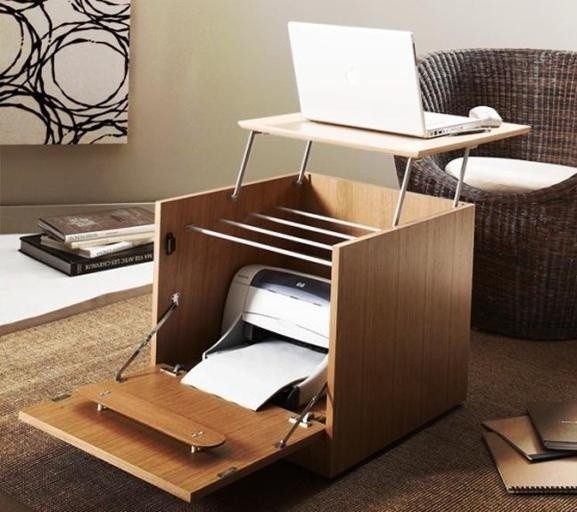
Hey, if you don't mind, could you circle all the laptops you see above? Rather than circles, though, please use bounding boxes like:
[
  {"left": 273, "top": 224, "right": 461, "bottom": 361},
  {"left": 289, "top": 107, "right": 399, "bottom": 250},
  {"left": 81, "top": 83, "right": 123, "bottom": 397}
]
[{"left": 285, "top": 20, "right": 476, "bottom": 139}]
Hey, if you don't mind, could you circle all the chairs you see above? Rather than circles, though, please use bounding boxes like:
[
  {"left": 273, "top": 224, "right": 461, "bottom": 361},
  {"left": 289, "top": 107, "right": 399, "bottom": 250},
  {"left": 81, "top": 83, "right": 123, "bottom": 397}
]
[{"left": 394, "top": 48, "right": 576, "bottom": 343}]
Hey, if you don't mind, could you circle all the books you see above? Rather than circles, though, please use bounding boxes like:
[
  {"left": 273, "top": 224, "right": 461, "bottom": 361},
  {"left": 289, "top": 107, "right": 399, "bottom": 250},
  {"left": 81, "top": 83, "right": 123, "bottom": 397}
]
[
  {"left": 18, "top": 206, "right": 154, "bottom": 276},
  {"left": 480, "top": 400, "right": 577, "bottom": 495}
]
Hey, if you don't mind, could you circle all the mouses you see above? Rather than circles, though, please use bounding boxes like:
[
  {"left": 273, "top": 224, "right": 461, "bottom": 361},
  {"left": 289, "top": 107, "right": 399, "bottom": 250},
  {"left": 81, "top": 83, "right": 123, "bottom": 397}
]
[{"left": 468, "top": 103, "right": 505, "bottom": 129}]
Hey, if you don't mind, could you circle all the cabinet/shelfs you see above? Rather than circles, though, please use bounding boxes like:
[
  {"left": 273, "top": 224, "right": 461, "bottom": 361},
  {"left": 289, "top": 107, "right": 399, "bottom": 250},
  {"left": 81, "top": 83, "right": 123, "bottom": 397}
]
[{"left": 19, "top": 104, "right": 531, "bottom": 504}]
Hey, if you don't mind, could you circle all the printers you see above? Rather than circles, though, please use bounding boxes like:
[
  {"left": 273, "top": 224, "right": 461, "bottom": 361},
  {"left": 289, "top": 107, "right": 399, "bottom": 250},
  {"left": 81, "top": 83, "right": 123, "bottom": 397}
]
[{"left": 183, "top": 261, "right": 331, "bottom": 412}]
